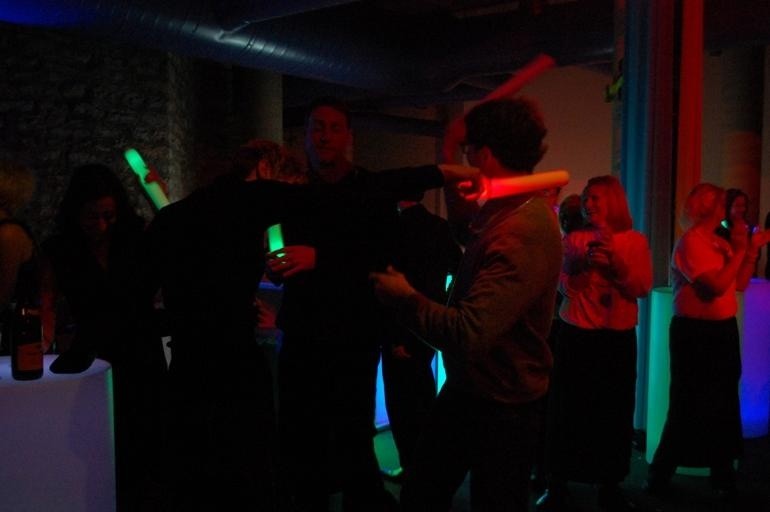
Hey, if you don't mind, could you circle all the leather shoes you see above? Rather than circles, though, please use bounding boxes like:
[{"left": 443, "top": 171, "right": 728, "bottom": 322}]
[
  {"left": 380, "top": 466, "right": 403, "bottom": 484},
  {"left": 535, "top": 489, "right": 550, "bottom": 512}
]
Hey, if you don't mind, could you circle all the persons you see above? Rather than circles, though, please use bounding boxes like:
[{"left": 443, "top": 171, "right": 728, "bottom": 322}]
[
  {"left": 640, "top": 181, "right": 768, "bottom": 497},
  {"left": 720, "top": 184, "right": 769, "bottom": 282}
]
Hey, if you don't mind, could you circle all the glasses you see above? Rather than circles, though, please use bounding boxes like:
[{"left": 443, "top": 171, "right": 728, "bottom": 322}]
[{"left": 461, "top": 138, "right": 480, "bottom": 154}]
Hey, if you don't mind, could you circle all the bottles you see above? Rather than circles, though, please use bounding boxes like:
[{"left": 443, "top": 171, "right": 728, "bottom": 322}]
[{"left": 8, "top": 261, "right": 45, "bottom": 380}]
